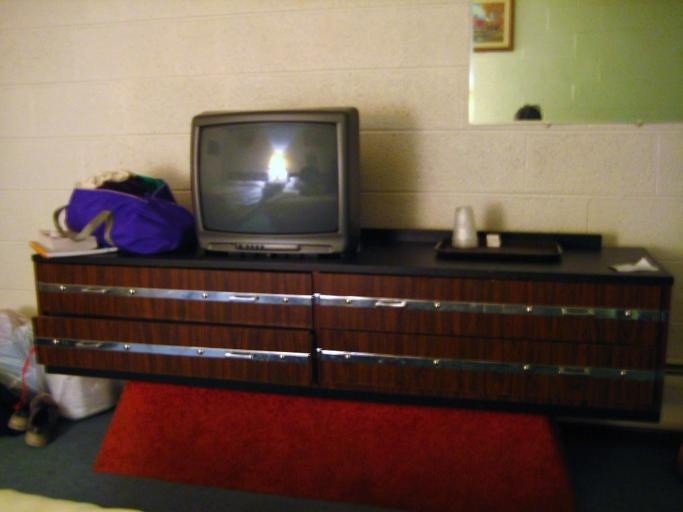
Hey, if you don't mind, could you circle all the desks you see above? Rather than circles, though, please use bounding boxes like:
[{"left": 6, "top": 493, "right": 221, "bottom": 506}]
[{"left": 30, "top": 247, "right": 675, "bottom": 423}]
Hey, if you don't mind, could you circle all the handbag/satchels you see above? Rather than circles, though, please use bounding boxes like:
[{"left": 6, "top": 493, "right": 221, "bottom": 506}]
[{"left": 53, "top": 176, "right": 196, "bottom": 258}]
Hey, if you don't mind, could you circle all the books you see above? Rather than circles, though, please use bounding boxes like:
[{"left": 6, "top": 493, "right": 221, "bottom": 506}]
[{"left": 26, "top": 226, "right": 121, "bottom": 261}]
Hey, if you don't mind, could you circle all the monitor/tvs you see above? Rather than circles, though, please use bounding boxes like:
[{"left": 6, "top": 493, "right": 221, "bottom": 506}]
[{"left": 191, "top": 107, "right": 360, "bottom": 255}]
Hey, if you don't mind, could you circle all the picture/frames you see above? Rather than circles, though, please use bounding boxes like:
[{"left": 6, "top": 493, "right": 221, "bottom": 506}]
[{"left": 471, "top": 1, "right": 514, "bottom": 51}]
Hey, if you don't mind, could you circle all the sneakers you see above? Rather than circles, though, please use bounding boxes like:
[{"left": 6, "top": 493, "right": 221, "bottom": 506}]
[
  {"left": 25, "top": 392, "right": 58, "bottom": 447},
  {"left": 8, "top": 401, "right": 30, "bottom": 432}
]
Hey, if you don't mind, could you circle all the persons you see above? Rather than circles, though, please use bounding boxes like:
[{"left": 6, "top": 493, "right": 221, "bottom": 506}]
[{"left": 514, "top": 102, "right": 543, "bottom": 122}]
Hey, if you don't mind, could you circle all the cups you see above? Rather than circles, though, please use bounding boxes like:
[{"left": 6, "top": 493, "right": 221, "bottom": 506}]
[{"left": 451, "top": 206, "right": 479, "bottom": 248}]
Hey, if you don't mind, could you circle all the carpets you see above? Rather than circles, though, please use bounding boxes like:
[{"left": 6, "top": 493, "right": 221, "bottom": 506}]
[{"left": 89, "top": 381, "right": 576, "bottom": 512}]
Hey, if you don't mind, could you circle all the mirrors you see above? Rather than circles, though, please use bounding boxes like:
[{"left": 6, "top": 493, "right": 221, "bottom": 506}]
[{"left": 469, "top": 0, "right": 682, "bottom": 123}]
